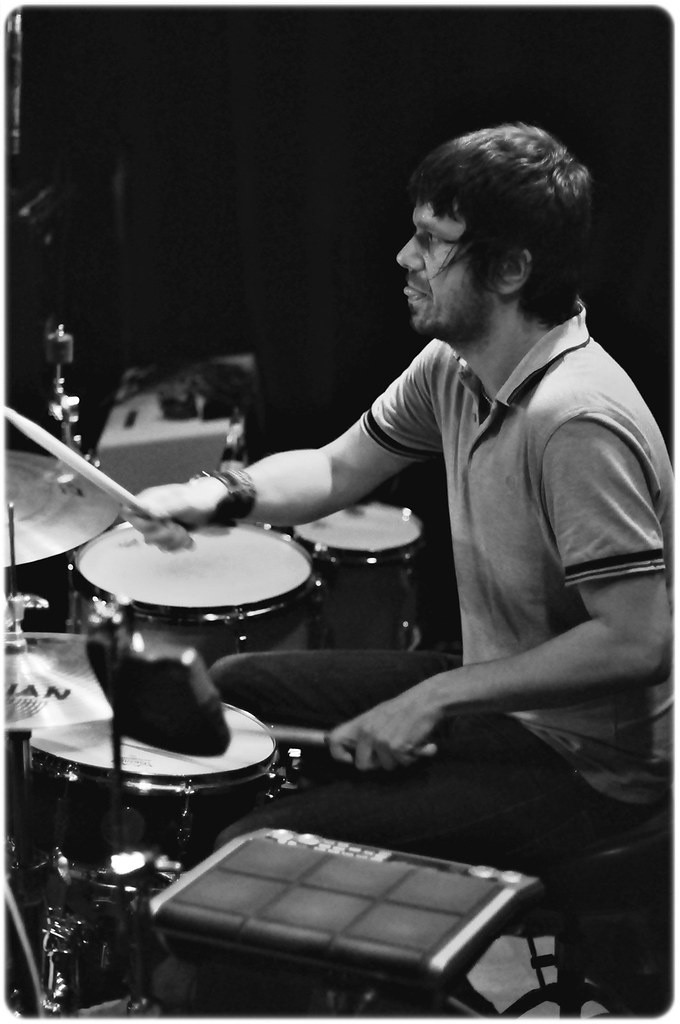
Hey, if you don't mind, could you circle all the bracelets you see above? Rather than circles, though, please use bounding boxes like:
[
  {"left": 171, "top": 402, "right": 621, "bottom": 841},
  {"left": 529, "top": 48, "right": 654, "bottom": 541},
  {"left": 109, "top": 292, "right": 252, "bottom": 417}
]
[{"left": 187, "top": 465, "right": 256, "bottom": 529}]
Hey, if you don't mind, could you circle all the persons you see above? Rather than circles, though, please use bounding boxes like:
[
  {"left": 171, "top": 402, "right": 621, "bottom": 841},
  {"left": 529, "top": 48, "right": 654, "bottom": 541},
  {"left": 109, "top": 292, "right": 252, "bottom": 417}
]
[{"left": 121, "top": 121, "right": 673, "bottom": 1015}]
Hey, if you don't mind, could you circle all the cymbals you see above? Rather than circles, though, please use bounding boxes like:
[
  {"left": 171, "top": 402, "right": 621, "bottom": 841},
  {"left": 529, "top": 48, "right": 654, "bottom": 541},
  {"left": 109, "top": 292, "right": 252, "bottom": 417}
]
[
  {"left": 4, "top": 625, "right": 117, "bottom": 734},
  {"left": 6, "top": 446, "right": 124, "bottom": 571}
]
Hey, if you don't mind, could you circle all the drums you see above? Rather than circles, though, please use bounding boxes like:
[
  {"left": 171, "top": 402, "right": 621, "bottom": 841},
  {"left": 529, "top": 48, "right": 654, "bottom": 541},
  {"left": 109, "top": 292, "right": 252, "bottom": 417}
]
[
  {"left": 24, "top": 695, "right": 287, "bottom": 1016},
  {"left": 67, "top": 509, "right": 330, "bottom": 675},
  {"left": 291, "top": 495, "right": 424, "bottom": 653}
]
